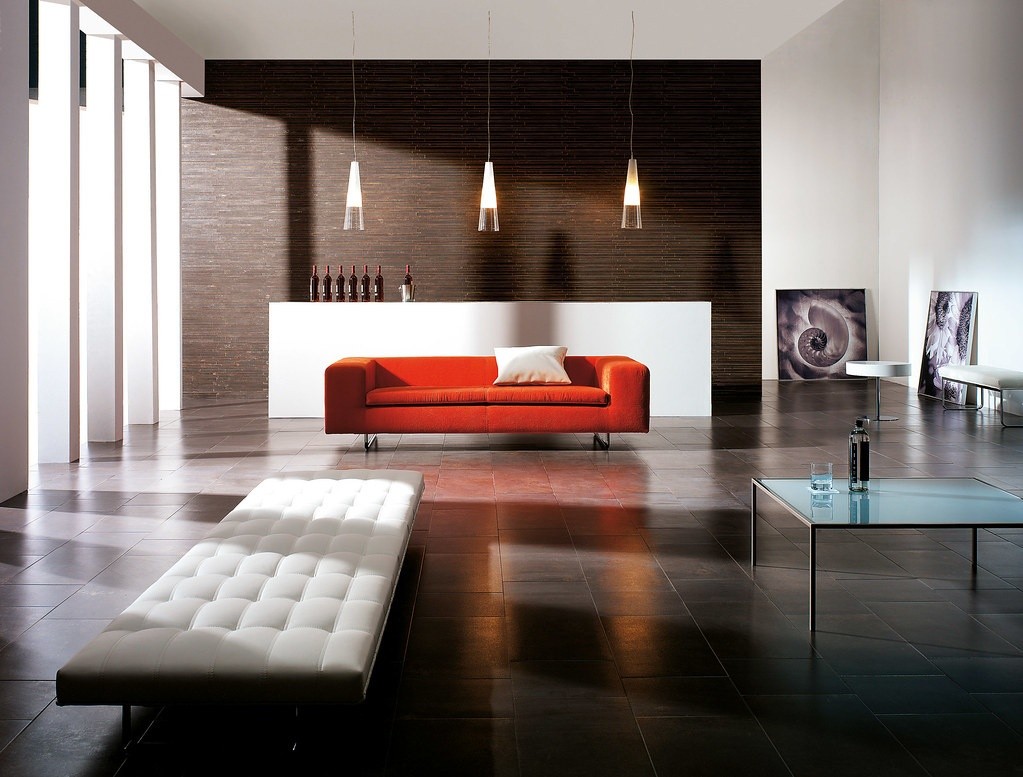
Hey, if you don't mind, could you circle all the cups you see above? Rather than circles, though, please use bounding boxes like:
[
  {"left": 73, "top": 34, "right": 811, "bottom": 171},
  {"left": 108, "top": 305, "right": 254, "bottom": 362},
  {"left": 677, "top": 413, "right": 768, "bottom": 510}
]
[
  {"left": 401, "top": 284, "right": 416, "bottom": 302},
  {"left": 810, "top": 463, "right": 833, "bottom": 490}
]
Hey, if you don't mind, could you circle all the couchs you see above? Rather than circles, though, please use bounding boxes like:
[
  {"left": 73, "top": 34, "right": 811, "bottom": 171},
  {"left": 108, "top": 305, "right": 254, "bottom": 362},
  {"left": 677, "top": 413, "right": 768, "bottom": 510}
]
[{"left": 326, "top": 356, "right": 651, "bottom": 450}]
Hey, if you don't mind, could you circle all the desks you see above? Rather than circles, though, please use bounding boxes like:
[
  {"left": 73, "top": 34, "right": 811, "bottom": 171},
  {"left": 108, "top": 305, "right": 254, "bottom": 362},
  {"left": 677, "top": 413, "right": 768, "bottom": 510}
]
[
  {"left": 846, "top": 361, "right": 912, "bottom": 421},
  {"left": 751, "top": 477, "right": 1023, "bottom": 632}
]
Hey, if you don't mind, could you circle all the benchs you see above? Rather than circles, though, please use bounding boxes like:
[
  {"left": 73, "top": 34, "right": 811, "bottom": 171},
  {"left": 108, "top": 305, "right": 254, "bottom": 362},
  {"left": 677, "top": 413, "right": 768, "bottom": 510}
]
[
  {"left": 55, "top": 469, "right": 426, "bottom": 755},
  {"left": 937, "top": 366, "right": 1023, "bottom": 427}
]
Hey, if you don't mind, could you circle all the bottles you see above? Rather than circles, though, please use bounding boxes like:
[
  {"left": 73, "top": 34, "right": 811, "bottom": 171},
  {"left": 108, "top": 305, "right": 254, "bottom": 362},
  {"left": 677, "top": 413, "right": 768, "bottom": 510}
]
[
  {"left": 349, "top": 265, "right": 358, "bottom": 302},
  {"left": 848, "top": 420, "right": 870, "bottom": 491},
  {"left": 361, "top": 265, "right": 371, "bottom": 302},
  {"left": 309, "top": 265, "right": 320, "bottom": 302},
  {"left": 403, "top": 265, "right": 414, "bottom": 285},
  {"left": 323, "top": 265, "right": 333, "bottom": 302},
  {"left": 335, "top": 265, "right": 346, "bottom": 302},
  {"left": 374, "top": 265, "right": 384, "bottom": 302}
]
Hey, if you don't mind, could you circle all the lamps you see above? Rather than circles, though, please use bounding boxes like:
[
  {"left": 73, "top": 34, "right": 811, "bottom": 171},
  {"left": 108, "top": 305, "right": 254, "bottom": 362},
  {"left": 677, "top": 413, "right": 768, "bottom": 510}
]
[
  {"left": 343, "top": 9, "right": 364, "bottom": 231},
  {"left": 622, "top": 11, "right": 642, "bottom": 228},
  {"left": 477, "top": 10, "right": 500, "bottom": 231}
]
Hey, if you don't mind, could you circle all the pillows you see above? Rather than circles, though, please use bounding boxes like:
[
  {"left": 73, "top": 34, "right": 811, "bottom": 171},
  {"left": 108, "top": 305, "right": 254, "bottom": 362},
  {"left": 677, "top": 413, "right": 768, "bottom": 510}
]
[{"left": 490, "top": 345, "right": 573, "bottom": 385}]
